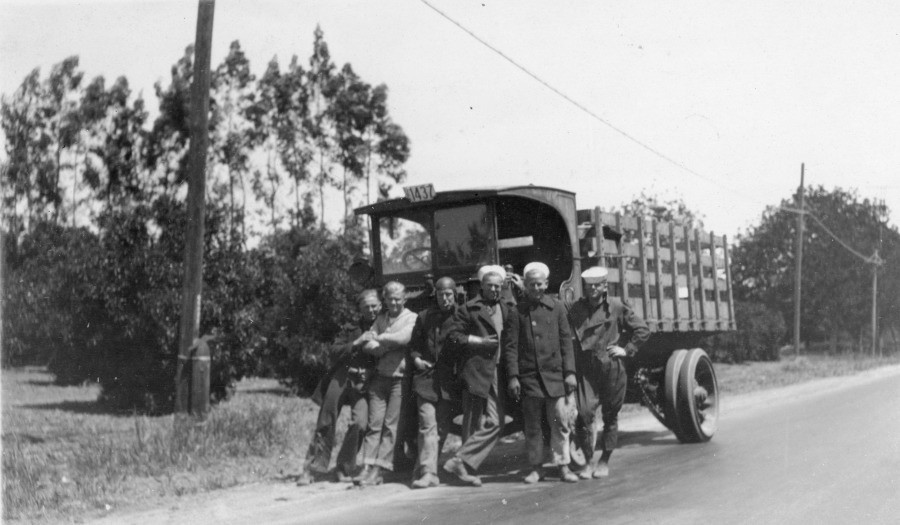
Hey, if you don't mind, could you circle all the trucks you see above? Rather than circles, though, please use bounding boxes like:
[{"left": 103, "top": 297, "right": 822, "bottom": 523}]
[{"left": 351, "top": 181, "right": 734, "bottom": 483}]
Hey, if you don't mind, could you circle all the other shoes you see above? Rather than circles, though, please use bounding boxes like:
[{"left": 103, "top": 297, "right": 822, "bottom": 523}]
[
  {"left": 560, "top": 466, "right": 579, "bottom": 482},
  {"left": 443, "top": 458, "right": 477, "bottom": 482},
  {"left": 411, "top": 472, "right": 439, "bottom": 488},
  {"left": 296, "top": 470, "right": 318, "bottom": 486},
  {"left": 593, "top": 465, "right": 609, "bottom": 478},
  {"left": 354, "top": 464, "right": 384, "bottom": 487},
  {"left": 580, "top": 463, "right": 593, "bottom": 479},
  {"left": 523, "top": 469, "right": 545, "bottom": 482},
  {"left": 337, "top": 470, "right": 352, "bottom": 482}
]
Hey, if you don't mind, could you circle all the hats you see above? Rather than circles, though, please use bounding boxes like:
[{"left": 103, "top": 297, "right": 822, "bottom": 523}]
[
  {"left": 581, "top": 266, "right": 608, "bottom": 284},
  {"left": 435, "top": 276, "right": 458, "bottom": 298}
]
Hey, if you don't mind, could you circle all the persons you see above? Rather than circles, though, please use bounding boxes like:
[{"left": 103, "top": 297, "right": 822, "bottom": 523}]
[
  {"left": 505, "top": 261, "right": 581, "bottom": 484},
  {"left": 445, "top": 263, "right": 512, "bottom": 488},
  {"left": 407, "top": 275, "right": 461, "bottom": 489},
  {"left": 348, "top": 280, "right": 420, "bottom": 485},
  {"left": 297, "top": 289, "right": 384, "bottom": 485},
  {"left": 508, "top": 265, "right": 652, "bottom": 482}
]
